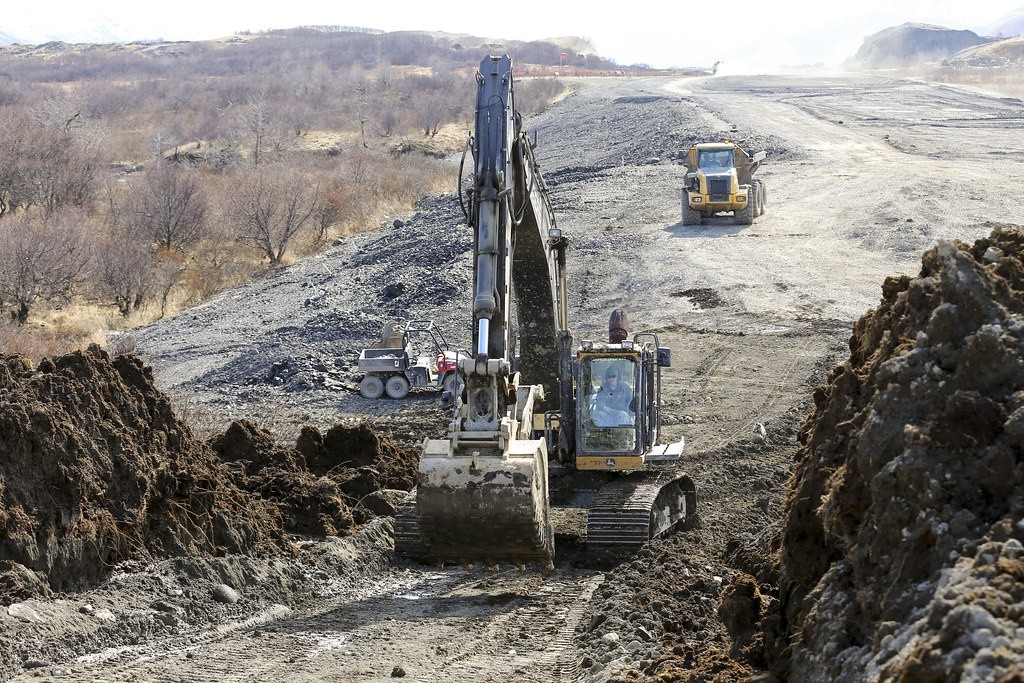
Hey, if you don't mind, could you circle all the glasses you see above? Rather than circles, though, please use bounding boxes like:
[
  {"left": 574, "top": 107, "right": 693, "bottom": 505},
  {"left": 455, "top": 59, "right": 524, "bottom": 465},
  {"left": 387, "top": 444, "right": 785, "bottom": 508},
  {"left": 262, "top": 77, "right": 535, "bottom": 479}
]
[
  {"left": 713, "top": 157, "right": 716, "bottom": 158},
  {"left": 608, "top": 375, "right": 618, "bottom": 379}
]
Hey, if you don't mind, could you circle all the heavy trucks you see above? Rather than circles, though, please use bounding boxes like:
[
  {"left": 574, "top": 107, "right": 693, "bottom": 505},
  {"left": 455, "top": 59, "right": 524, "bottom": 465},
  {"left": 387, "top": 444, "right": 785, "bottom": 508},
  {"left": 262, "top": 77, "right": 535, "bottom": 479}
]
[{"left": 682, "top": 141, "right": 768, "bottom": 224}]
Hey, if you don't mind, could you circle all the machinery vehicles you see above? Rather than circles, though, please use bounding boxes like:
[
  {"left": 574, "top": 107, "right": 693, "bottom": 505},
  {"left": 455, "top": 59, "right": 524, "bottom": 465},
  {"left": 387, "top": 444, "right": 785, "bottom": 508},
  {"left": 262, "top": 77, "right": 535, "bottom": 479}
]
[
  {"left": 360, "top": 320, "right": 468, "bottom": 399},
  {"left": 394, "top": 54, "right": 695, "bottom": 568}
]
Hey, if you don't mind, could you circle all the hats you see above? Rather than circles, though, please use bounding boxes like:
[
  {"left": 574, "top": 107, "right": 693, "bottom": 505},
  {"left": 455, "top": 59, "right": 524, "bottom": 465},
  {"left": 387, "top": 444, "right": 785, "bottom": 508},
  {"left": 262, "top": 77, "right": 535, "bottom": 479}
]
[{"left": 605, "top": 367, "right": 621, "bottom": 381}]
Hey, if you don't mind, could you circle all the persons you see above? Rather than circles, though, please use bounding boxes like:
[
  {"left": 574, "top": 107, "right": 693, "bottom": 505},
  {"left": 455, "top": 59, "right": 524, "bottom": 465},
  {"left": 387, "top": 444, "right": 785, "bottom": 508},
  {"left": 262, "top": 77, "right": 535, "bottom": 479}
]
[{"left": 594, "top": 367, "right": 634, "bottom": 424}]
